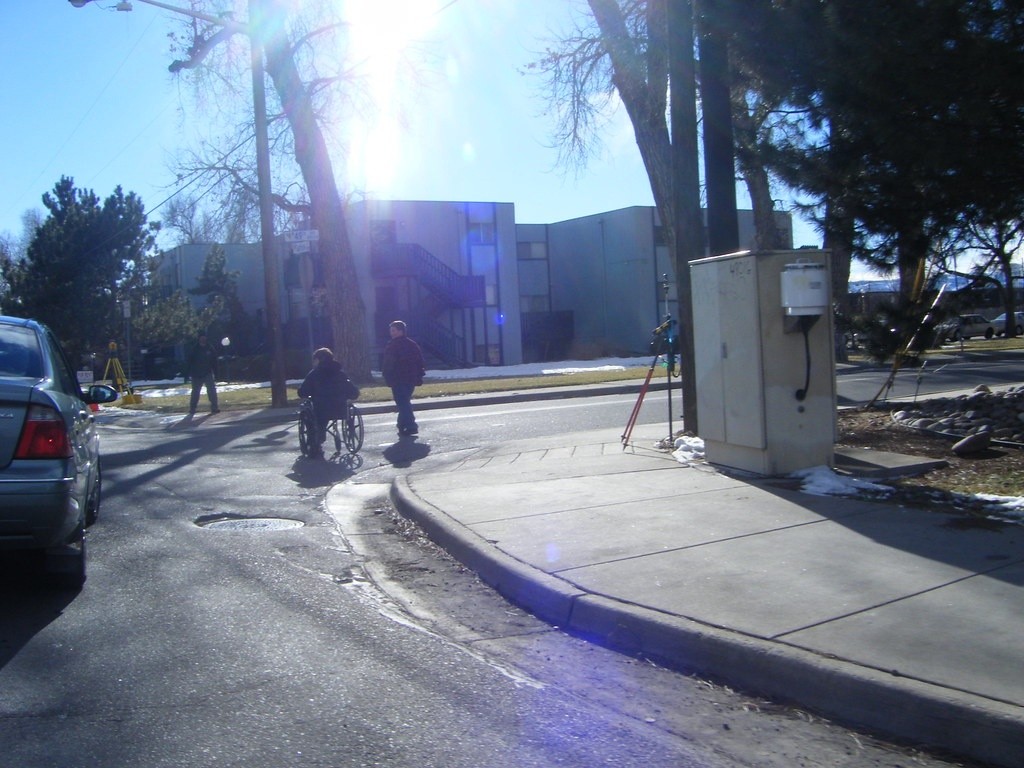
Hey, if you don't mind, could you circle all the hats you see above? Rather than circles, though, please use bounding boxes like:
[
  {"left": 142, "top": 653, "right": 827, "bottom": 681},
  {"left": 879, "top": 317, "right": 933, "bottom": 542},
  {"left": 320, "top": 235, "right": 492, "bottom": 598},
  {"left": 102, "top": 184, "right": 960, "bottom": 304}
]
[{"left": 199, "top": 329, "right": 208, "bottom": 337}]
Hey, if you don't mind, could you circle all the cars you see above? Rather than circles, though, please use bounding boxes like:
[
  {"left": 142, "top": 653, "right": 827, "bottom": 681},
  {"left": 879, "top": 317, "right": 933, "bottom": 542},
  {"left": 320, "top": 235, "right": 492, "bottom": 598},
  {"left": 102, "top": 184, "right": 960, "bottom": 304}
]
[
  {"left": 992, "top": 312, "right": 1024, "bottom": 336},
  {"left": 840, "top": 320, "right": 896, "bottom": 347},
  {"left": 0, "top": 314, "right": 118, "bottom": 589},
  {"left": 933, "top": 314, "right": 998, "bottom": 342}
]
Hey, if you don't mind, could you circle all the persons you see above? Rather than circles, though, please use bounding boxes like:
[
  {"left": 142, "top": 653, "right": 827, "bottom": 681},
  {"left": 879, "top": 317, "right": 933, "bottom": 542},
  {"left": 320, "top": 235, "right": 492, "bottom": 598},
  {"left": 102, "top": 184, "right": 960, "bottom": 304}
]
[
  {"left": 297, "top": 347, "right": 361, "bottom": 444},
  {"left": 187, "top": 332, "right": 221, "bottom": 415},
  {"left": 382, "top": 321, "right": 427, "bottom": 436}
]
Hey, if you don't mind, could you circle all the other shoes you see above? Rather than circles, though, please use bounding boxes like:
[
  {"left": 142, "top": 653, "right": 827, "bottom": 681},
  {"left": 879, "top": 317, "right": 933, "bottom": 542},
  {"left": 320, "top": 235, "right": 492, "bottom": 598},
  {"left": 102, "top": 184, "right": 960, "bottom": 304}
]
[
  {"left": 212, "top": 408, "right": 221, "bottom": 413},
  {"left": 189, "top": 411, "right": 195, "bottom": 416},
  {"left": 397, "top": 422, "right": 406, "bottom": 429},
  {"left": 402, "top": 428, "right": 418, "bottom": 434}
]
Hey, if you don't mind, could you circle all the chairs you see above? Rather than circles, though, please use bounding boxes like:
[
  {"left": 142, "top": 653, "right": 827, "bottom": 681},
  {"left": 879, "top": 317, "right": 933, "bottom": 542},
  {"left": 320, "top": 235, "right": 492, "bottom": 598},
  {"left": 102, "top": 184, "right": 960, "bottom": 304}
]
[{"left": 0, "top": 342, "right": 43, "bottom": 377}]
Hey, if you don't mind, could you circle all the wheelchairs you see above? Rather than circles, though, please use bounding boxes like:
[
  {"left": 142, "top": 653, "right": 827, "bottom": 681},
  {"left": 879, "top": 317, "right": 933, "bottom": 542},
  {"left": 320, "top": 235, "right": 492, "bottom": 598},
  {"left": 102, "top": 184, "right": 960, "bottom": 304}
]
[{"left": 298, "top": 382, "right": 364, "bottom": 458}]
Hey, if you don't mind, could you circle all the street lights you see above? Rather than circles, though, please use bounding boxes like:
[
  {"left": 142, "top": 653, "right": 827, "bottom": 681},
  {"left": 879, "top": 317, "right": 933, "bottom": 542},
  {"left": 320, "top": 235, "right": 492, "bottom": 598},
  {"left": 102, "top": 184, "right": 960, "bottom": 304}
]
[
  {"left": 69, "top": 1, "right": 289, "bottom": 409},
  {"left": 116, "top": 293, "right": 134, "bottom": 395}
]
